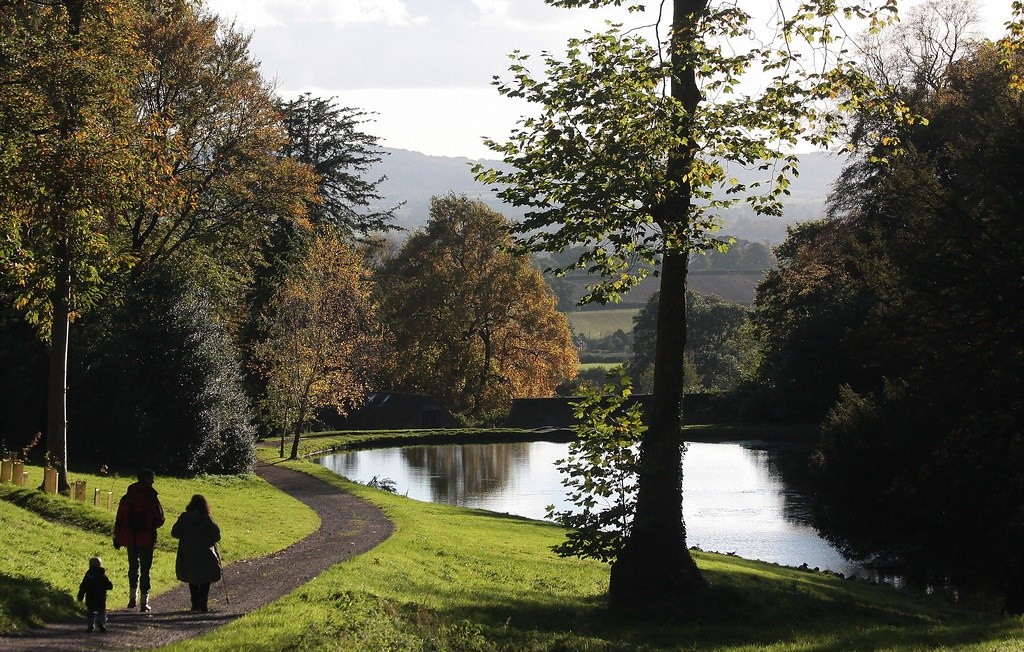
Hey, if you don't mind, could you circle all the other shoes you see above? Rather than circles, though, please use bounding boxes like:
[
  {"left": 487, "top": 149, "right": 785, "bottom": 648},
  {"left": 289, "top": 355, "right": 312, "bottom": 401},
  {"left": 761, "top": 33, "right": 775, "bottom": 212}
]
[
  {"left": 86, "top": 628, "right": 94, "bottom": 633},
  {"left": 141, "top": 603, "right": 151, "bottom": 612},
  {"left": 191, "top": 606, "right": 199, "bottom": 611},
  {"left": 97, "top": 622, "right": 107, "bottom": 633},
  {"left": 127, "top": 600, "right": 136, "bottom": 608},
  {"left": 200, "top": 603, "right": 208, "bottom": 613}
]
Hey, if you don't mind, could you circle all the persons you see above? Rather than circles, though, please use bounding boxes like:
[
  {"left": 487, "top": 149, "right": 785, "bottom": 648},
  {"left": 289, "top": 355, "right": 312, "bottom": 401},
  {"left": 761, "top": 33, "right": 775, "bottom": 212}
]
[
  {"left": 171, "top": 494, "right": 222, "bottom": 612},
  {"left": 78, "top": 558, "right": 113, "bottom": 631},
  {"left": 113, "top": 469, "right": 165, "bottom": 610}
]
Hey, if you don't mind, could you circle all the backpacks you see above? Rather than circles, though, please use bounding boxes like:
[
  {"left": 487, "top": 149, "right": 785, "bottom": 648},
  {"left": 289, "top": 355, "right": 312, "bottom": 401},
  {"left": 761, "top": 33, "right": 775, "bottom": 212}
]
[{"left": 126, "top": 499, "right": 151, "bottom": 533}]
